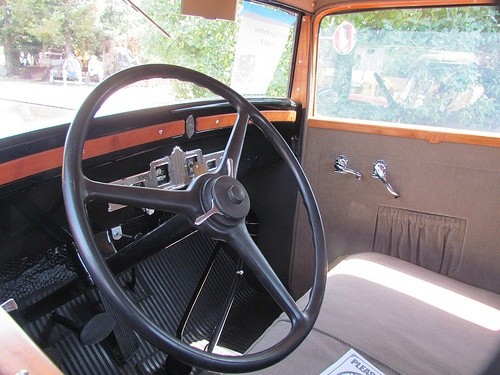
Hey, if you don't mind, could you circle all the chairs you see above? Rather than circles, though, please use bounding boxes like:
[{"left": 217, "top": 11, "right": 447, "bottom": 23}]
[{"left": 235, "top": 252, "right": 500, "bottom": 374}]
